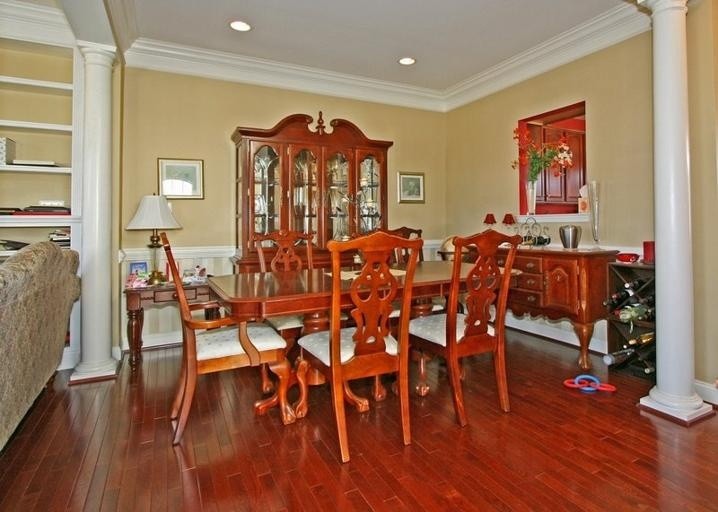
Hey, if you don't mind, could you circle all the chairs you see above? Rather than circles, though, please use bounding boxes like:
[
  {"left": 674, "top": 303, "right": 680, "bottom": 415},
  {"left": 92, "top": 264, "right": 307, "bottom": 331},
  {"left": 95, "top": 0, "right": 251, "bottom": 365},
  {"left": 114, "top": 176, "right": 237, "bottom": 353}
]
[
  {"left": 296, "top": 229, "right": 424, "bottom": 465},
  {"left": 249, "top": 226, "right": 317, "bottom": 365},
  {"left": 351, "top": 225, "right": 424, "bottom": 301},
  {"left": 157, "top": 230, "right": 298, "bottom": 443},
  {"left": 408, "top": 229, "right": 523, "bottom": 427}
]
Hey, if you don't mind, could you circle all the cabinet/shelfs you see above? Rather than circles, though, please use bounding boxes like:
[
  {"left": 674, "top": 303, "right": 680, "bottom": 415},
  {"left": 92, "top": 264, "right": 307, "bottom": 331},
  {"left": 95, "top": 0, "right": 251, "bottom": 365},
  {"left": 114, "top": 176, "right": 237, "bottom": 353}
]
[
  {"left": 230, "top": 112, "right": 394, "bottom": 271},
  {"left": 460, "top": 242, "right": 621, "bottom": 376},
  {"left": 526, "top": 118, "right": 585, "bottom": 213},
  {"left": 0, "top": 37, "right": 83, "bottom": 368}
]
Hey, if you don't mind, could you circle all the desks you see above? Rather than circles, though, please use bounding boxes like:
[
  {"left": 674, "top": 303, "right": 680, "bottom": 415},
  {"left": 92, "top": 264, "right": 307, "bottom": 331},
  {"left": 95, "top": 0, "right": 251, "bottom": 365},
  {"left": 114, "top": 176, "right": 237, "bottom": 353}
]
[
  {"left": 123, "top": 279, "right": 222, "bottom": 369},
  {"left": 206, "top": 260, "right": 522, "bottom": 418}
]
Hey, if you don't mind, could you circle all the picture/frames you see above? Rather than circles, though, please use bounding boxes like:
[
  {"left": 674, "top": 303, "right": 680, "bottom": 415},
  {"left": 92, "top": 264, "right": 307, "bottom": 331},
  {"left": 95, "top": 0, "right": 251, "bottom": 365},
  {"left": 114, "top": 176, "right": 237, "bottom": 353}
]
[
  {"left": 158, "top": 157, "right": 204, "bottom": 199},
  {"left": 128, "top": 261, "right": 148, "bottom": 276},
  {"left": 397, "top": 172, "right": 424, "bottom": 203}
]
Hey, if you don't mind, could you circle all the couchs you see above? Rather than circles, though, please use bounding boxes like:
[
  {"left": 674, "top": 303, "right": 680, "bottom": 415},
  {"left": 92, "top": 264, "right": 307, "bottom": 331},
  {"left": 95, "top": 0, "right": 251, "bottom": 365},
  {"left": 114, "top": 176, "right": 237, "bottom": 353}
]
[{"left": 0, "top": 244, "right": 82, "bottom": 454}]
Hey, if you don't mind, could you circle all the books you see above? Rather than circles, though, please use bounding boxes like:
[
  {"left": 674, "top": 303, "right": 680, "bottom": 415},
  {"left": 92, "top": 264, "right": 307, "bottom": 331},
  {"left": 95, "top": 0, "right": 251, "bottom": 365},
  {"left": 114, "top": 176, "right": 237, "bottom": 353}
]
[
  {"left": 0, "top": 205, "right": 71, "bottom": 216},
  {"left": 47, "top": 229, "right": 72, "bottom": 249},
  {"left": 0, "top": 136, "right": 17, "bottom": 167},
  {"left": 0, "top": 238, "right": 30, "bottom": 256},
  {"left": 12, "top": 160, "right": 66, "bottom": 169}
]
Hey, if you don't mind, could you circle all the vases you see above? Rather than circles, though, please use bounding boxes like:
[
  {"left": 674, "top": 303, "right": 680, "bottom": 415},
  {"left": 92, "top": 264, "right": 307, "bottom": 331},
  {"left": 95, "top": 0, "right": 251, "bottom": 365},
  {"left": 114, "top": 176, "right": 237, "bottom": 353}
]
[{"left": 525, "top": 180, "right": 537, "bottom": 215}]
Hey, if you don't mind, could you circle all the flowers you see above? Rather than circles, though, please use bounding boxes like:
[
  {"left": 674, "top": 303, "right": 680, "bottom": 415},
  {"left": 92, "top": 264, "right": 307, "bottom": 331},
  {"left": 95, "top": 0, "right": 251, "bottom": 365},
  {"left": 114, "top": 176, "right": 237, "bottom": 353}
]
[{"left": 509, "top": 119, "right": 574, "bottom": 187}]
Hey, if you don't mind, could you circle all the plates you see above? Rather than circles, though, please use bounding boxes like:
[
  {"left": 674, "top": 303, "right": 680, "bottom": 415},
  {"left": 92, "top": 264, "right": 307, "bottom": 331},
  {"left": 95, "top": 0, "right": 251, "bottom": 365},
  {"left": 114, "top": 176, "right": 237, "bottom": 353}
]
[{"left": 616, "top": 253, "right": 640, "bottom": 261}]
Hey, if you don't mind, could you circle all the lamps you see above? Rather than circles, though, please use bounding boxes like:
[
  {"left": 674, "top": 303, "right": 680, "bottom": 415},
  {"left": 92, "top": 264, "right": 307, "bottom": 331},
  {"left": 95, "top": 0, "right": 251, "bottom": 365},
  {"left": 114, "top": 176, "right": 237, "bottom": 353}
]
[
  {"left": 483, "top": 214, "right": 496, "bottom": 231},
  {"left": 125, "top": 193, "right": 182, "bottom": 285},
  {"left": 503, "top": 213, "right": 515, "bottom": 238}
]
